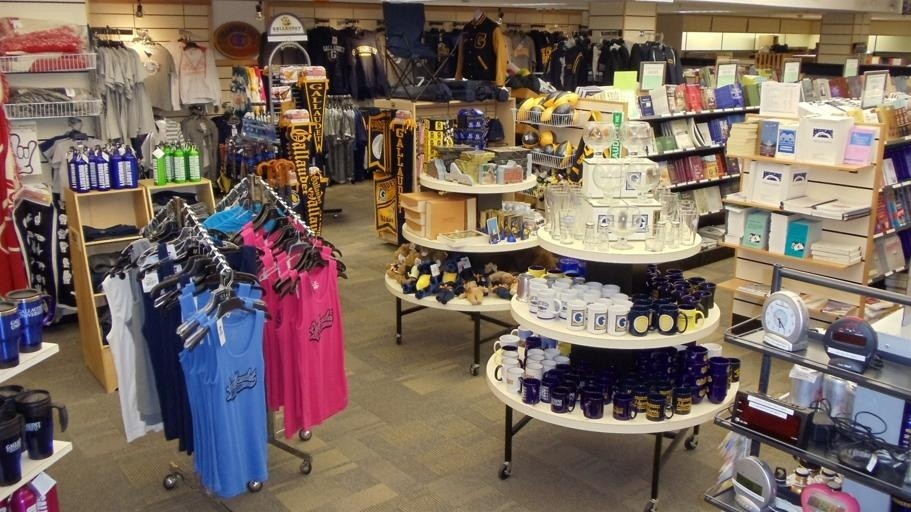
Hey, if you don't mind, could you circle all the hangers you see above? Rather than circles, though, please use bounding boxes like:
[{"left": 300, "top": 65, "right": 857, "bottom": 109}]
[
  {"left": 136, "top": 30, "right": 162, "bottom": 49},
  {"left": 121, "top": 176, "right": 348, "bottom": 356},
  {"left": 175, "top": 31, "right": 208, "bottom": 56}
]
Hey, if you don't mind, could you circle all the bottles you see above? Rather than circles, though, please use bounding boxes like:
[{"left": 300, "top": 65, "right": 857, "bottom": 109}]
[
  {"left": 0, "top": 474, "right": 60, "bottom": 512},
  {"left": 66, "top": 141, "right": 139, "bottom": 194},
  {"left": 151, "top": 139, "right": 201, "bottom": 187}
]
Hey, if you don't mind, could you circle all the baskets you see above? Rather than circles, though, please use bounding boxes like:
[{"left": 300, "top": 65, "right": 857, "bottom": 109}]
[
  {"left": 515, "top": 108, "right": 574, "bottom": 128},
  {"left": 531, "top": 150, "right": 577, "bottom": 169},
  {"left": 3, "top": 93, "right": 103, "bottom": 121},
  {"left": 0, "top": 52, "right": 98, "bottom": 72}
]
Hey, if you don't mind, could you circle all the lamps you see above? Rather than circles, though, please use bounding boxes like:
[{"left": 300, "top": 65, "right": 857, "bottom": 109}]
[
  {"left": 253, "top": 0, "right": 267, "bottom": 20},
  {"left": 135, "top": 0, "right": 144, "bottom": 17}
]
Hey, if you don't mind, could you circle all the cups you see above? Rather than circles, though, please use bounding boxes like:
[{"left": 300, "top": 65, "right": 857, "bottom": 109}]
[
  {"left": 492, "top": 325, "right": 741, "bottom": 423},
  {"left": 0, "top": 288, "right": 56, "bottom": 371},
  {"left": 515, "top": 258, "right": 717, "bottom": 338},
  {"left": 543, "top": 183, "right": 700, "bottom": 253},
  {"left": 0, "top": 384, "right": 69, "bottom": 487}
]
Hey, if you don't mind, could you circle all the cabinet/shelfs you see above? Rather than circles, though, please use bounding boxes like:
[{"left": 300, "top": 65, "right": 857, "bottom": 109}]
[
  {"left": 384, "top": 165, "right": 544, "bottom": 375},
  {"left": 0, "top": 341, "right": 70, "bottom": 509},
  {"left": 2, "top": 49, "right": 105, "bottom": 120},
  {"left": 63, "top": 174, "right": 220, "bottom": 395},
  {"left": 374, "top": 68, "right": 761, "bottom": 272},
  {"left": 261, "top": 62, "right": 330, "bottom": 135},
  {"left": 705, "top": 72, "right": 911, "bottom": 512},
  {"left": 486, "top": 157, "right": 741, "bottom": 510}
]
